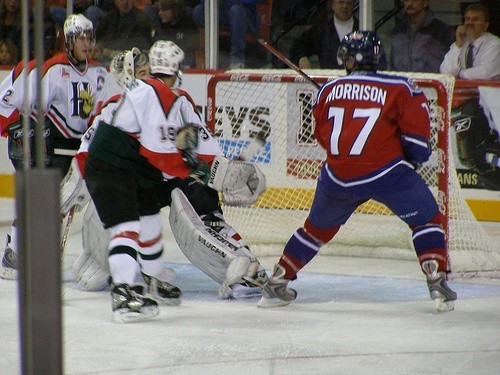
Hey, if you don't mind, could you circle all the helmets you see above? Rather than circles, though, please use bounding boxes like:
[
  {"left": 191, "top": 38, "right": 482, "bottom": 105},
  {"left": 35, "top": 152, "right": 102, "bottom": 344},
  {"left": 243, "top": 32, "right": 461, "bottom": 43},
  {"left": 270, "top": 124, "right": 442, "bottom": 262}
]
[
  {"left": 109, "top": 47, "right": 147, "bottom": 92},
  {"left": 63, "top": 13, "right": 96, "bottom": 46},
  {"left": 337, "top": 31, "right": 387, "bottom": 71},
  {"left": 148, "top": 40, "right": 185, "bottom": 75}
]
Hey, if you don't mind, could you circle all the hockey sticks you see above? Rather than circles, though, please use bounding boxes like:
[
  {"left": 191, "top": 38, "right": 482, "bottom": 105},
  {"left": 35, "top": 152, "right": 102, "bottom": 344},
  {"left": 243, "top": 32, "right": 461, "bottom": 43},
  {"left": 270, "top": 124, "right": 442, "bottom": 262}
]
[
  {"left": 257, "top": 37, "right": 421, "bottom": 169},
  {"left": 54, "top": 148, "right": 78, "bottom": 156}
]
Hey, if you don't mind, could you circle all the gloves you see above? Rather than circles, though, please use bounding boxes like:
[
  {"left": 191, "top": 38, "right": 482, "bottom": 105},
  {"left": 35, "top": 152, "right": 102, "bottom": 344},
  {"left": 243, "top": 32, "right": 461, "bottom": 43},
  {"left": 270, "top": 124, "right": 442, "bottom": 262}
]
[{"left": 7, "top": 120, "right": 23, "bottom": 161}]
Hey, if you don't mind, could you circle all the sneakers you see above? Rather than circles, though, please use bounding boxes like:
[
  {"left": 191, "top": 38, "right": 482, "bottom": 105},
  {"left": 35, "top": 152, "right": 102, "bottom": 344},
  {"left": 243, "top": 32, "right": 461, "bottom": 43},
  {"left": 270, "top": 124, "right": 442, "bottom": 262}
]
[
  {"left": 110, "top": 283, "right": 160, "bottom": 323},
  {"left": 0, "top": 233, "right": 18, "bottom": 280},
  {"left": 229, "top": 269, "right": 270, "bottom": 299},
  {"left": 132, "top": 270, "right": 183, "bottom": 306},
  {"left": 421, "top": 260, "right": 457, "bottom": 312},
  {"left": 257, "top": 262, "right": 298, "bottom": 307}
]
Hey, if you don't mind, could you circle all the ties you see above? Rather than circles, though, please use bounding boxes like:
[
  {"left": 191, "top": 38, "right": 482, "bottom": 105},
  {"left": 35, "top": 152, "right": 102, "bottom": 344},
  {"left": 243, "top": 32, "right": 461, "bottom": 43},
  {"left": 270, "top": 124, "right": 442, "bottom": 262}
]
[{"left": 466, "top": 44, "right": 474, "bottom": 68}]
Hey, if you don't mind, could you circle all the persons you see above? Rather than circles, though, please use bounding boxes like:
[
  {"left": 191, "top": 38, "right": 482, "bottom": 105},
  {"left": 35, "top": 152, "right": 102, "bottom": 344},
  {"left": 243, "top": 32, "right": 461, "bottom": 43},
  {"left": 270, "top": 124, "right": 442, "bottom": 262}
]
[
  {"left": 440, "top": 3, "right": 500, "bottom": 80},
  {"left": 390, "top": 0, "right": 455, "bottom": 72},
  {"left": 76, "top": 41, "right": 211, "bottom": 322},
  {"left": 73, "top": 0, "right": 260, "bottom": 70},
  {"left": 257, "top": 30, "right": 458, "bottom": 309},
  {"left": 0, "top": 0, "right": 66, "bottom": 65},
  {"left": 270, "top": 0, "right": 359, "bottom": 70},
  {"left": 0, "top": 15, "right": 109, "bottom": 278},
  {"left": 61, "top": 47, "right": 267, "bottom": 299}
]
